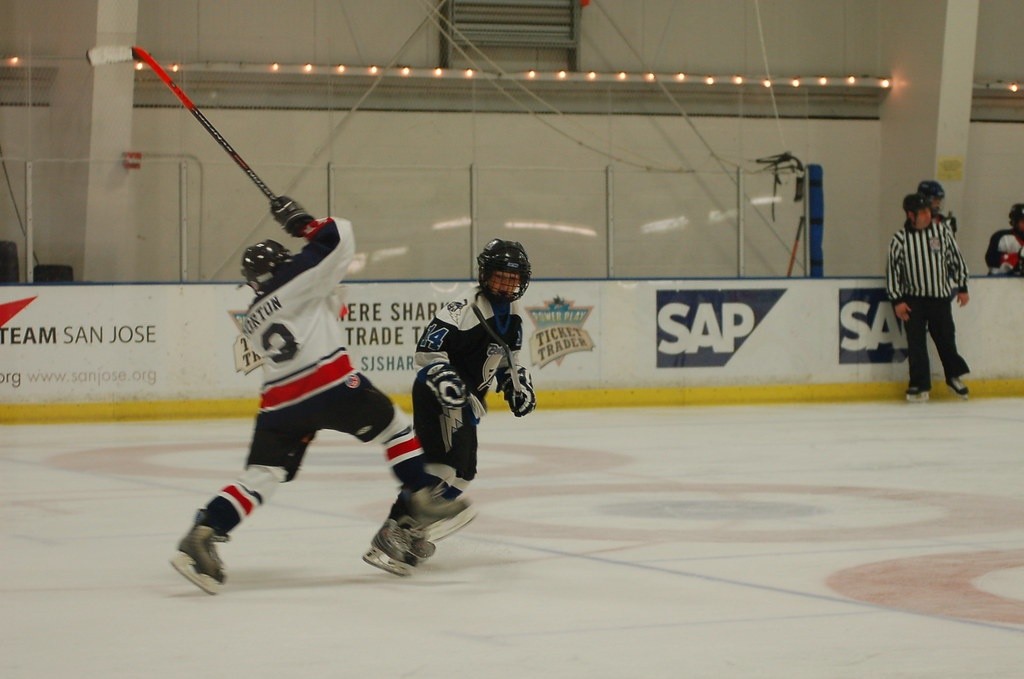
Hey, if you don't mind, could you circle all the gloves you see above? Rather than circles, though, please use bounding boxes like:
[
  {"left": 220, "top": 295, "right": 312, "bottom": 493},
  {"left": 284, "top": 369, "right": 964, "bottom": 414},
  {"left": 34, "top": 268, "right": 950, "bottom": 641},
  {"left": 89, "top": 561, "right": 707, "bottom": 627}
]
[
  {"left": 270, "top": 195, "right": 313, "bottom": 237},
  {"left": 502, "top": 365, "right": 537, "bottom": 418},
  {"left": 1001, "top": 253, "right": 1019, "bottom": 269},
  {"left": 426, "top": 364, "right": 470, "bottom": 410}
]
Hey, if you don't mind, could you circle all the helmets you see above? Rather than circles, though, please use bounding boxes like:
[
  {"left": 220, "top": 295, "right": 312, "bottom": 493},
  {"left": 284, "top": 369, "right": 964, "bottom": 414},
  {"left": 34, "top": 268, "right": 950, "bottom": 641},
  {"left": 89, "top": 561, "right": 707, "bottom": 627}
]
[
  {"left": 242, "top": 240, "right": 290, "bottom": 290},
  {"left": 1009, "top": 204, "right": 1024, "bottom": 217},
  {"left": 477, "top": 238, "right": 530, "bottom": 287},
  {"left": 903, "top": 193, "right": 932, "bottom": 211},
  {"left": 917, "top": 180, "right": 945, "bottom": 199}
]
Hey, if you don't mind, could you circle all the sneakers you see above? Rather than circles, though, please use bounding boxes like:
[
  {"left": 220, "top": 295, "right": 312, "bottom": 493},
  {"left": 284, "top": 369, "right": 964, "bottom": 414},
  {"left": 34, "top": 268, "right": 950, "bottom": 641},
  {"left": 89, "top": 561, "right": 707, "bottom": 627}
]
[
  {"left": 409, "top": 477, "right": 480, "bottom": 544},
  {"left": 168, "top": 510, "right": 230, "bottom": 595},
  {"left": 358, "top": 516, "right": 434, "bottom": 575},
  {"left": 945, "top": 376, "right": 968, "bottom": 399},
  {"left": 905, "top": 386, "right": 931, "bottom": 401}
]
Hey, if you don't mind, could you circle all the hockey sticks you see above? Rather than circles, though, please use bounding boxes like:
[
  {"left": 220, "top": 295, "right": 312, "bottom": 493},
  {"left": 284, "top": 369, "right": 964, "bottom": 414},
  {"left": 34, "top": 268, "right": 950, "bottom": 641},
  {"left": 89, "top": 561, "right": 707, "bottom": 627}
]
[
  {"left": 84, "top": 43, "right": 276, "bottom": 217},
  {"left": 471, "top": 303, "right": 525, "bottom": 394}
]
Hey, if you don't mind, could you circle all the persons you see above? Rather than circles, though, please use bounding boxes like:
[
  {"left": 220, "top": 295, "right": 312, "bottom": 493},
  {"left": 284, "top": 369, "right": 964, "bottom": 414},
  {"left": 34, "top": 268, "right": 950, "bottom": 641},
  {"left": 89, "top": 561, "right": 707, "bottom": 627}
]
[
  {"left": 985, "top": 203, "right": 1024, "bottom": 277},
  {"left": 370, "top": 238, "right": 536, "bottom": 564},
  {"left": 886, "top": 180, "right": 970, "bottom": 395},
  {"left": 177, "top": 196, "right": 470, "bottom": 585}
]
